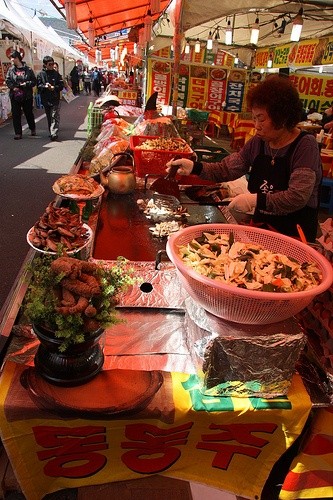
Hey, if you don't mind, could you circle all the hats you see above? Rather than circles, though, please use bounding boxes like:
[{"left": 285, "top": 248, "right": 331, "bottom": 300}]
[{"left": 10, "top": 51, "right": 22, "bottom": 61}]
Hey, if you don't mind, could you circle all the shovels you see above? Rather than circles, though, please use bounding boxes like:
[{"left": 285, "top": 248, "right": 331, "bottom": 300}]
[{"left": 148, "top": 155, "right": 182, "bottom": 200}]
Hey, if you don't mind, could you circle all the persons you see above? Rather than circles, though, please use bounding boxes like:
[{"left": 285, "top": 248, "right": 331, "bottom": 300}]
[
  {"left": 5, "top": 50, "right": 37, "bottom": 139},
  {"left": 37, "top": 55, "right": 64, "bottom": 141},
  {"left": 167, "top": 76, "right": 323, "bottom": 243},
  {"left": 69, "top": 66, "right": 134, "bottom": 98}
]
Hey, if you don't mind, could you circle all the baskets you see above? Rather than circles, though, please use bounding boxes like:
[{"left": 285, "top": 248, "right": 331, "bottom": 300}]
[{"left": 166, "top": 224, "right": 333, "bottom": 325}]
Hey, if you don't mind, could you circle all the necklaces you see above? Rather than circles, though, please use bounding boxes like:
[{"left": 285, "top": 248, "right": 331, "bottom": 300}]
[{"left": 270, "top": 142, "right": 280, "bottom": 165}]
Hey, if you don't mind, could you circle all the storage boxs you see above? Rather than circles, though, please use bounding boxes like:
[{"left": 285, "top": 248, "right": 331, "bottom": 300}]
[{"left": 128, "top": 134, "right": 194, "bottom": 177}]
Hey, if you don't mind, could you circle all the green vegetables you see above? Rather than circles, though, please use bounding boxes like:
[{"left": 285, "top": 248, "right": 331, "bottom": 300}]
[{"left": 178, "top": 231, "right": 322, "bottom": 294}]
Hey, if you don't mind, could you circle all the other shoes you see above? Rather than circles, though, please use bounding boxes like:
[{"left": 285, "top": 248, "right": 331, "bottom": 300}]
[
  {"left": 48, "top": 133, "right": 58, "bottom": 141},
  {"left": 31, "top": 129, "right": 36, "bottom": 135},
  {"left": 14, "top": 134, "right": 22, "bottom": 139}
]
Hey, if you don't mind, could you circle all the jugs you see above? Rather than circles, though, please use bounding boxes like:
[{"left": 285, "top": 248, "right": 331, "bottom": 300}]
[{"left": 98, "top": 152, "right": 137, "bottom": 194}]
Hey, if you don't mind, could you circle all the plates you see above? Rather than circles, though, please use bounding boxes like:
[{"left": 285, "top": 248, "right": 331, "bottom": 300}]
[
  {"left": 151, "top": 61, "right": 170, "bottom": 74},
  {"left": 211, "top": 69, "right": 226, "bottom": 80},
  {"left": 26, "top": 221, "right": 93, "bottom": 255},
  {"left": 52, "top": 181, "right": 105, "bottom": 199}
]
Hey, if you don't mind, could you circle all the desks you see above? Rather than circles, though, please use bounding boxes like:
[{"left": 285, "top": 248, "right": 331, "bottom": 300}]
[{"left": 208, "top": 110, "right": 333, "bottom": 181}]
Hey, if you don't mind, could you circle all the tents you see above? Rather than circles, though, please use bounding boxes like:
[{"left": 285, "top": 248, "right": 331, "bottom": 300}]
[{"left": 0, "top": 0, "right": 89, "bottom": 123}]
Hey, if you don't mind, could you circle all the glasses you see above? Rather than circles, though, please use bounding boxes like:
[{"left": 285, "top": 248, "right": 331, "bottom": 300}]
[{"left": 49, "top": 63, "right": 54, "bottom": 66}]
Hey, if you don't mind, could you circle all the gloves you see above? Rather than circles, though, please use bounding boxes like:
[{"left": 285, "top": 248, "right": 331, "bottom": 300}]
[
  {"left": 222, "top": 193, "right": 257, "bottom": 213},
  {"left": 166, "top": 158, "right": 194, "bottom": 176}
]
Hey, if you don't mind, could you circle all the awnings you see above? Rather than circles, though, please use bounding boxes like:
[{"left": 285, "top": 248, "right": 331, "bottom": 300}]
[{"left": 49, "top": 0, "right": 172, "bottom": 66}]
